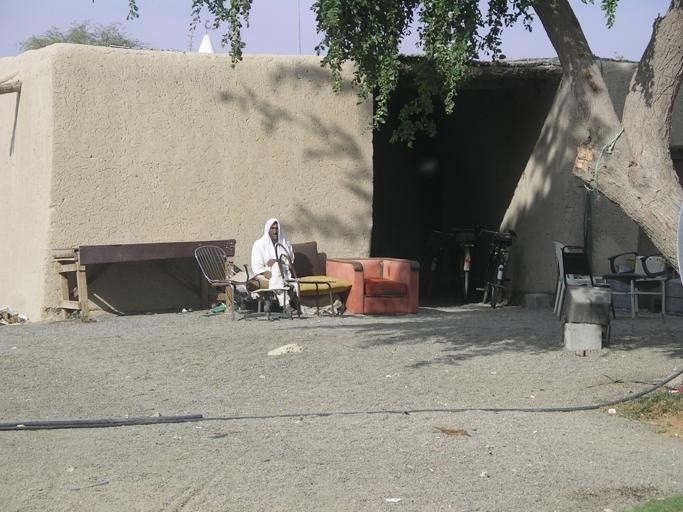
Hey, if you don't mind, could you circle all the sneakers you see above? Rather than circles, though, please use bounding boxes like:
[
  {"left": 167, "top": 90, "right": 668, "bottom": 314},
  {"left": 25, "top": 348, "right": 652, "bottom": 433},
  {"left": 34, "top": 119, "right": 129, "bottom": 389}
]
[{"left": 283, "top": 305, "right": 298, "bottom": 315}]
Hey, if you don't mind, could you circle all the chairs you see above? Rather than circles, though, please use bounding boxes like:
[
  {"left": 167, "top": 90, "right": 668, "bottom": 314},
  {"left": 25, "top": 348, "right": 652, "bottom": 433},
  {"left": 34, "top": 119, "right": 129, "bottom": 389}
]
[
  {"left": 552, "top": 239, "right": 673, "bottom": 323},
  {"left": 194, "top": 240, "right": 420, "bottom": 322}
]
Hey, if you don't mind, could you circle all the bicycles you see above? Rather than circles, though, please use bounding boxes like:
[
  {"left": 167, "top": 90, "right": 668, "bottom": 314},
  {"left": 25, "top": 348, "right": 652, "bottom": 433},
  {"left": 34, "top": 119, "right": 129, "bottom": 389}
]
[{"left": 427, "top": 228, "right": 512, "bottom": 308}]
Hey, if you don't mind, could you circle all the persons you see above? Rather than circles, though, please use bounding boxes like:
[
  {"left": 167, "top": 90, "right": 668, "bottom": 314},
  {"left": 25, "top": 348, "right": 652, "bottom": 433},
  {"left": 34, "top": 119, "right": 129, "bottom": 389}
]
[{"left": 250, "top": 217, "right": 302, "bottom": 317}]
